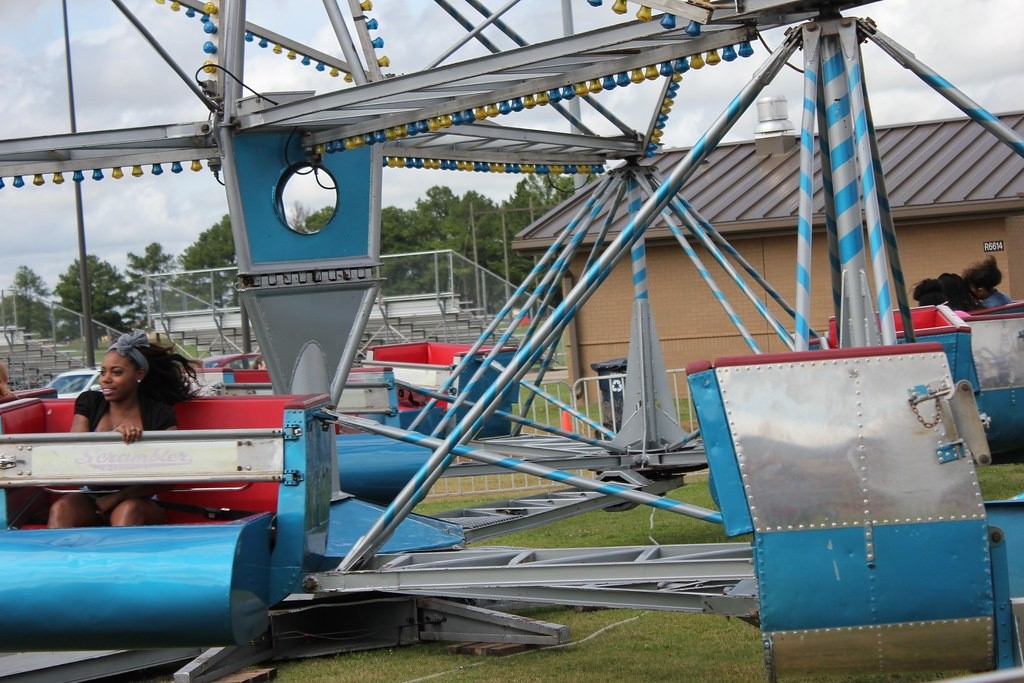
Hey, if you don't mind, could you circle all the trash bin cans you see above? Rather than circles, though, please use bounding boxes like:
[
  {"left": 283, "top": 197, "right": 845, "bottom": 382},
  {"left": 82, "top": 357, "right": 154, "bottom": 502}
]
[{"left": 590, "top": 358, "right": 628, "bottom": 440}]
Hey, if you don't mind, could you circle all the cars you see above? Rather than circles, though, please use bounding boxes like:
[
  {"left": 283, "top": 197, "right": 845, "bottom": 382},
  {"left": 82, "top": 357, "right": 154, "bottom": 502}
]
[
  {"left": 191, "top": 353, "right": 264, "bottom": 370},
  {"left": 42, "top": 366, "right": 109, "bottom": 400}
]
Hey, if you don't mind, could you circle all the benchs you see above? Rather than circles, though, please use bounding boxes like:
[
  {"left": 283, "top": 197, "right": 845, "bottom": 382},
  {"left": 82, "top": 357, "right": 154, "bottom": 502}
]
[
  {"left": 150, "top": 292, "right": 518, "bottom": 358},
  {"left": 0, "top": 324, "right": 89, "bottom": 390}
]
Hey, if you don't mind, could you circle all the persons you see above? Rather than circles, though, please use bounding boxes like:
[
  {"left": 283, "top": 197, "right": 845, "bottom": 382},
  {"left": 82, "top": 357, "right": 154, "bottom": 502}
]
[
  {"left": 912, "top": 256, "right": 1012, "bottom": 318},
  {"left": 48, "top": 331, "right": 202, "bottom": 527}
]
